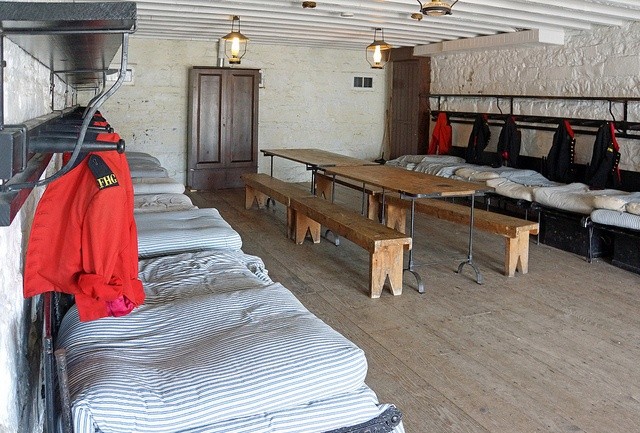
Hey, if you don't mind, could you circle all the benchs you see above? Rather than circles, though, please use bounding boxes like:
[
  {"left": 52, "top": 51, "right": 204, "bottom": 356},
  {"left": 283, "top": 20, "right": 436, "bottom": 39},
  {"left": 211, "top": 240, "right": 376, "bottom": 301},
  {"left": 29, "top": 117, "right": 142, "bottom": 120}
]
[
  {"left": 239, "top": 173, "right": 319, "bottom": 240},
  {"left": 377, "top": 192, "right": 541, "bottom": 278},
  {"left": 290, "top": 196, "right": 414, "bottom": 299},
  {"left": 313, "top": 165, "right": 398, "bottom": 223}
]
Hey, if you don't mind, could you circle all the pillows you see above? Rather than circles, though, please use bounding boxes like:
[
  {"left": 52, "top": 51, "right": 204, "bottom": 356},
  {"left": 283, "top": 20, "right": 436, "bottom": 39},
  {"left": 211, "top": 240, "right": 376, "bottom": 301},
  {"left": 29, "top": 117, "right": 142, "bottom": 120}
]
[
  {"left": 456, "top": 166, "right": 499, "bottom": 182},
  {"left": 494, "top": 178, "right": 537, "bottom": 203},
  {"left": 591, "top": 194, "right": 640, "bottom": 215}
]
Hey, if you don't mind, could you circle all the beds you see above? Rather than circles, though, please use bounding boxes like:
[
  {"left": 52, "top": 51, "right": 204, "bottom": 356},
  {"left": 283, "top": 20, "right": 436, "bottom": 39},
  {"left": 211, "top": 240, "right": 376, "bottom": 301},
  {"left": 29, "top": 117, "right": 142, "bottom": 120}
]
[
  {"left": 133, "top": 206, "right": 243, "bottom": 261},
  {"left": 127, "top": 156, "right": 170, "bottom": 179},
  {"left": 129, "top": 175, "right": 185, "bottom": 195},
  {"left": 384, "top": 153, "right": 468, "bottom": 175},
  {"left": 532, "top": 181, "right": 628, "bottom": 264},
  {"left": 486, "top": 168, "right": 566, "bottom": 243},
  {"left": 124, "top": 150, "right": 162, "bottom": 165},
  {"left": 448, "top": 164, "right": 519, "bottom": 195},
  {"left": 50, "top": 280, "right": 406, "bottom": 433},
  {"left": 137, "top": 250, "right": 276, "bottom": 304},
  {"left": 580, "top": 190, "right": 640, "bottom": 275},
  {"left": 133, "top": 190, "right": 197, "bottom": 212}
]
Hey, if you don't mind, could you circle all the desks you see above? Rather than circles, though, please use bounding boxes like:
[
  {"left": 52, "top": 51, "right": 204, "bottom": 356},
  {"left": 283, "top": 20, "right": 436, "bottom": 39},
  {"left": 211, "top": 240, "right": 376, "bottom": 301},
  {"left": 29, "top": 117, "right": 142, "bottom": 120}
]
[
  {"left": 261, "top": 147, "right": 381, "bottom": 216},
  {"left": 317, "top": 165, "right": 497, "bottom": 294}
]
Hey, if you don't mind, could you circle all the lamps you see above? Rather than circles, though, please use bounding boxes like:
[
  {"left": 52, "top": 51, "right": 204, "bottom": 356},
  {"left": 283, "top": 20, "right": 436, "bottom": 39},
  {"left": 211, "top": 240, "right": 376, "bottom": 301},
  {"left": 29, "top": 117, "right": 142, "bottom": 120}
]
[
  {"left": 365, "top": 27, "right": 392, "bottom": 70},
  {"left": 221, "top": 15, "right": 248, "bottom": 64},
  {"left": 417, "top": 0, "right": 458, "bottom": 16}
]
